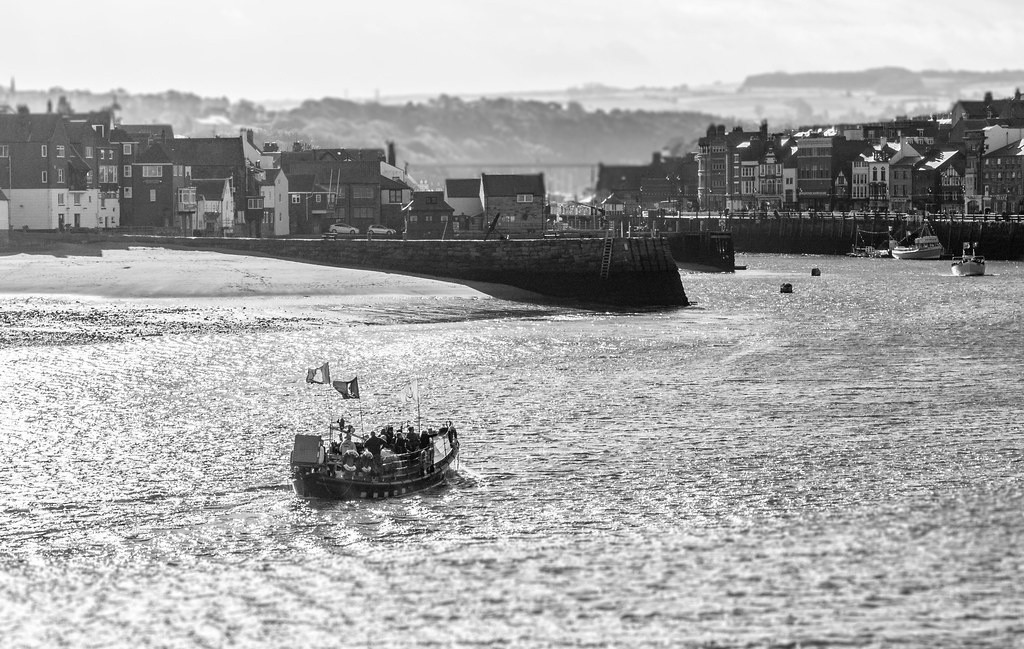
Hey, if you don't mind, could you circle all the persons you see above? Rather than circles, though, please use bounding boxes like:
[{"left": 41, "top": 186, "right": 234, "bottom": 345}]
[
  {"left": 339, "top": 434, "right": 358, "bottom": 477},
  {"left": 364, "top": 431, "right": 386, "bottom": 482},
  {"left": 378, "top": 425, "right": 437, "bottom": 471}
]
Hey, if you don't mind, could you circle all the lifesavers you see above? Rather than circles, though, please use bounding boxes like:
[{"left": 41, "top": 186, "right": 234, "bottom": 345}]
[
  {"left": 359, "top": 450, "right": 374, "bottom": 474},
  {"left": 341, "top": 450, "right": 359, "bottom": 472}
]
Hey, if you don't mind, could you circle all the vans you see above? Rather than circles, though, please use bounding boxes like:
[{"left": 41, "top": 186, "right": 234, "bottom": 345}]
[{"left": 949, "top": 210, "right": 959, "bottom": 214}]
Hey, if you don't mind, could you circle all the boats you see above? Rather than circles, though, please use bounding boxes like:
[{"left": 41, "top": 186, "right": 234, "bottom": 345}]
[
  {"left": 288, "top": 362, "right": 460, "bottom": 501},
  {"left": 846, "top": 224, "right": 906, "bottom": 258},
  {"left": 891, "top": 218, "right": 943, "bottom": 260},
  {"left": 949, "top": 241, "right": 986, "bottom": 277}
]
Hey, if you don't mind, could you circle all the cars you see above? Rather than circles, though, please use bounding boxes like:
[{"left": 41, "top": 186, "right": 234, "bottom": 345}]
[
  {"left": 328, "top": 223, "right": 360, "bottom": 234},
  {"left": 368, "top": 224, "right": 397, "bottom": 236}
]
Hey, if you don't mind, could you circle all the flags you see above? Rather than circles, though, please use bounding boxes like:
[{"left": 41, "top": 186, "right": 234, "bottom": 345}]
[
  {"left": 398, "top": 379, "right": 419, "bottom": 406},
  {"left": 332, "top": 377, "right": 359, "bottom": 399},
  {"left": 306, "top": 362, "right": 331, "bottom": 385}
]
